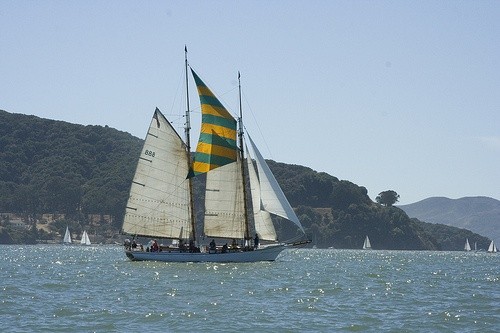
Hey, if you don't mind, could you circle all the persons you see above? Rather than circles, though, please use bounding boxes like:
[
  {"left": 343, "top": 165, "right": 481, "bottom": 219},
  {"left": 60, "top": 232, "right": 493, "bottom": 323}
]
[
  {"left": 221, "top": 243, "right": 228, "bottom": 253},
  {"left": 253, "top": 233, "right": 259, "bottom": 251},
  {"left": 232, "top": 238, "right": 237, "bottom": 248},
  {"left": 146, "top": 240, "right": 158, "bottom": 252},
  {"left": 210, "top": 239, "right": 216, "bottom": 253},
  {"left": 132, "top": 241, "right": 138, "bottom": 248}
]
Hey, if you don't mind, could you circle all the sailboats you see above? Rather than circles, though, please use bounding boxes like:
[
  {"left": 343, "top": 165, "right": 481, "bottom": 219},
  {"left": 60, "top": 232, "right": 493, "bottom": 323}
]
[
  {"left": 79, "top": 228, "right": 92, "bottom": 246},
  {"left": 118, "top": 44, "right": 313, "bottom": 263},
  {"left": 361, "top": 235, "right": 373, "bottom": 250},
  {"left": 487, "top": 240, "right": 497, "bottom": 253},
  {"left": 62, "top": 226, "right": 74, "bottom": 246},
  {"left": 463, "top": 238, "right": 472, "bottom": 251}
]
